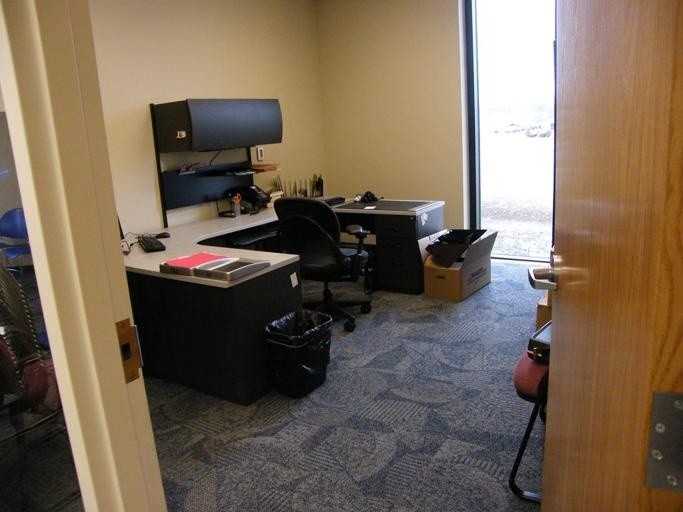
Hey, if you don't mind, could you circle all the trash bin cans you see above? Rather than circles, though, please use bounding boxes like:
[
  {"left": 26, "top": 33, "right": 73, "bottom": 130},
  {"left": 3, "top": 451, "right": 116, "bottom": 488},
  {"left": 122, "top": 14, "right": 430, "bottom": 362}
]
[{"left": 265, "top": 309, "right": 333, "bottom": 397}]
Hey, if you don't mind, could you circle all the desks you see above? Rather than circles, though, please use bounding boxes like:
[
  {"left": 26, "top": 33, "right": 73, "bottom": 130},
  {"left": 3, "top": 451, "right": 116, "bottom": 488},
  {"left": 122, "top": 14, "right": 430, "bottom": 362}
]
[{"left": 122, "top": 197, "right": 445, "bottom": 407}]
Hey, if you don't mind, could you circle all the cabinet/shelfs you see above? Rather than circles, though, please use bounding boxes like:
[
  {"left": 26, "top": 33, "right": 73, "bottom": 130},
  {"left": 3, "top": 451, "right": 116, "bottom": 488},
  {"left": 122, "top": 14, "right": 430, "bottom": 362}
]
[
  {"left": 151, "top": 98, "right": 282, "bottom": 229},
  {"left": 527, "top": 0, "right": 683, "bottom": 512}
]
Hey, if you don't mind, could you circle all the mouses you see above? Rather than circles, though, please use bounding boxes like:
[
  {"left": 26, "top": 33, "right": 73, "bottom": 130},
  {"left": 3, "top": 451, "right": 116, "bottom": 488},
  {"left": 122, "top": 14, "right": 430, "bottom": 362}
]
[{"left": 156, "top": 232, "right": 171, "bottom": 239}]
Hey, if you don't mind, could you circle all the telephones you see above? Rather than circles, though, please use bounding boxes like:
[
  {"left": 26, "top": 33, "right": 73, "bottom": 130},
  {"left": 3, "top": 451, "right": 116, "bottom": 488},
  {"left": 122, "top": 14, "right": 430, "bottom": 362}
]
[{"left": 245, "top": 185, "right": 271, "bottom": 206}]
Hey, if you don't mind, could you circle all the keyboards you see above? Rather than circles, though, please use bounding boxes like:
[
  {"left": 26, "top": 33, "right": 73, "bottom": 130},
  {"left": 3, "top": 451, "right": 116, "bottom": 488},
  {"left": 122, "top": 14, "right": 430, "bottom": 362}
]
[{"left": 138, "top": 235, "right": 166, "bottom": 253}]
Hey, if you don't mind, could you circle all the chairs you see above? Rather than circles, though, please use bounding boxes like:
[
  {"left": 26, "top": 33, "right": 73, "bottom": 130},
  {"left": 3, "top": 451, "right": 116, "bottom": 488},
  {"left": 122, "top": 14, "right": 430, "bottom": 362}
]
[
  {"left": 0, "top": 208, "right": 33, "bottom": 275},
  {"left": 273, "top": 197, "right": 371, "bottom": 332},
  {"left": 0, "top": 337, "right": 66, "bottom": 449}
]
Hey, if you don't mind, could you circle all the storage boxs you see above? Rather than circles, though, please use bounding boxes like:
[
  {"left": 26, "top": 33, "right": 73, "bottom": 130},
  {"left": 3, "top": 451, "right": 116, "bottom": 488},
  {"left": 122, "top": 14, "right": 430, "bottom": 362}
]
[{"left": 418, "top": 229, "right": 498, "bottom": 301}]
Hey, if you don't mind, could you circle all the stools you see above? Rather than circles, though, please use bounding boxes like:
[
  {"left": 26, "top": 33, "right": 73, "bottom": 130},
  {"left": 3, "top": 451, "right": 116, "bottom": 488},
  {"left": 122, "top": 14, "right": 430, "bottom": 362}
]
[{"left": 508, "top": 352, "right": 549, "bottom": 504}]
